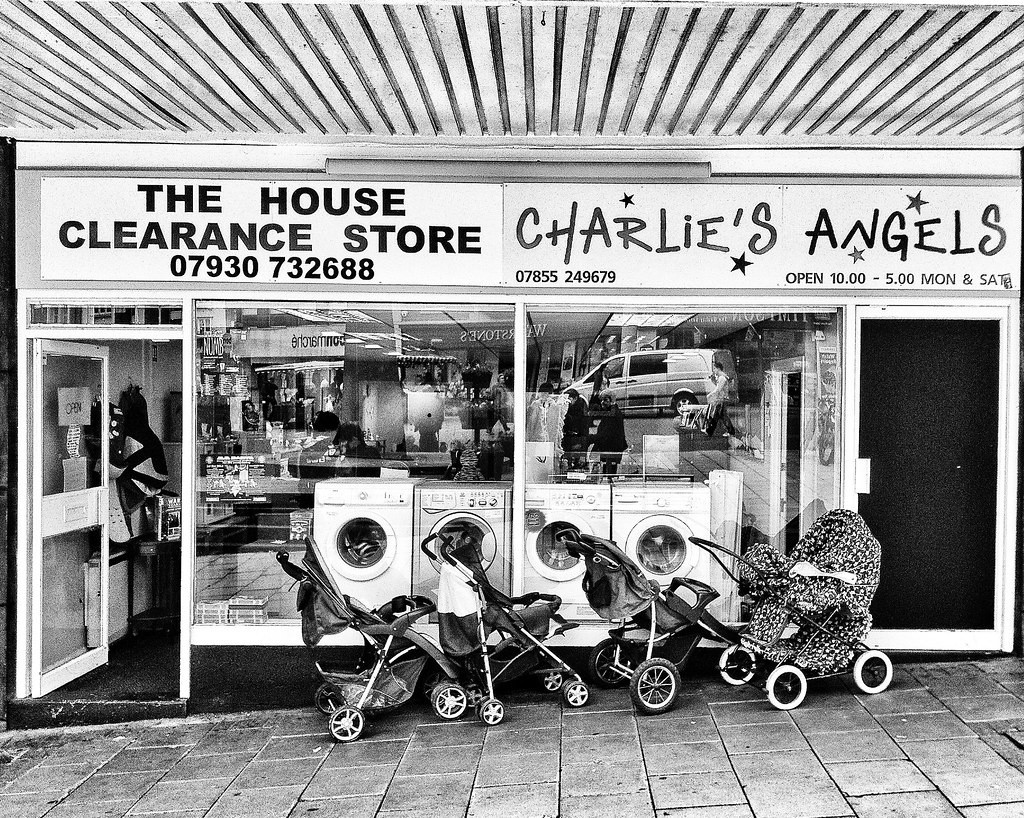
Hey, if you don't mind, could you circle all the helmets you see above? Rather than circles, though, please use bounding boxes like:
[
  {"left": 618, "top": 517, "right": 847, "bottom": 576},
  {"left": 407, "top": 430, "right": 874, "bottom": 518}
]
[{"left": 598, "top": 389, "right": 617, "bottom": 405}]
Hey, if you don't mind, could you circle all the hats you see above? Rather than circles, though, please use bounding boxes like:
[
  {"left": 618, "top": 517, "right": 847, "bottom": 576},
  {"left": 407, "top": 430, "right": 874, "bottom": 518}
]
[{"left": 538, "top": 383, "right": 553, "bottom": 393}]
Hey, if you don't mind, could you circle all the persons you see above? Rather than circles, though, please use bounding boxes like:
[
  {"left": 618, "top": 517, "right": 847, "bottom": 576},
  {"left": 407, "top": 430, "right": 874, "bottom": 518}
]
[
  {"left": 486, "top": 373, "right": 511, "bottom": 435},
  {"left": 296, "top": 411, "right": 382, "bottom": 510},
  {"left": 589, "top": 389, "right": 629, "bottom": 478},
  {"left": 703, "top": 362, "right": 735, "bottom": 438},
  {"left": 562, "top": 388, "right": 589, "bottom": 467}
]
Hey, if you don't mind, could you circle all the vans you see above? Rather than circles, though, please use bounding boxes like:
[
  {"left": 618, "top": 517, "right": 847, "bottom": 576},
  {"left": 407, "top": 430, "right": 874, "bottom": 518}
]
[{"left": 561, "top": 347, "right": 742, "bottom": 415}]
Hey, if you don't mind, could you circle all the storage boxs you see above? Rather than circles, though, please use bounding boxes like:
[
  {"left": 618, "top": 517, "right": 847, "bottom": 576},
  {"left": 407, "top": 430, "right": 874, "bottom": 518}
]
[
  {"left": 194, "top": 596, "right": 268, "bottom": 625},
  {"left": 289, "top": 510, "right": 312, "bottom": 542}
]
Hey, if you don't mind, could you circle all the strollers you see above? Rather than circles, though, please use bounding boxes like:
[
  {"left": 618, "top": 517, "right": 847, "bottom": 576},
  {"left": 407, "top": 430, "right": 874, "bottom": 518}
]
[
  {"left": 277, "top": 534, "right": 471, "bottom": 744},
  {"left": 689, "top": 508, "right": 896, "bottom": 710},
  {"left": 421, "top": 532, "right": 592, "bottom": 726},
  {"left": 671, "top": 402, "right": 710, "bottom": 439},
  {"left": 555, "top": 527, "right": 746, "bottom": 714}
]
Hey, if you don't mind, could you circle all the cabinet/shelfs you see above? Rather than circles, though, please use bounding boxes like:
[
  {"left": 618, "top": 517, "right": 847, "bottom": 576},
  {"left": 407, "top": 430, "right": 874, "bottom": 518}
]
[{"left": 126, "top": 541, "right": 179, "bottom": 637}]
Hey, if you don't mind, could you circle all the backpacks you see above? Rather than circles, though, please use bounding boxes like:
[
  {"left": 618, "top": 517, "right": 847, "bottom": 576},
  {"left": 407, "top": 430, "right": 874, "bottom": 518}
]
[{"left": 721, "top": 374, "right": 734, "bottom": 392}]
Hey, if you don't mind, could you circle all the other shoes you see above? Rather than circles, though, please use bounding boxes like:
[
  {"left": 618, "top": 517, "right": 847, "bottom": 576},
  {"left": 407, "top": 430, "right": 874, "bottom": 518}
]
[
  {"left": 723, "top": 432, "right": 733, "bottom": 437},
  {"left": 700, "top": 429, "right": 709, "bottom": 436},
  {"left": 486, "top": 430, "right": 494, "bottom": 435}
]
